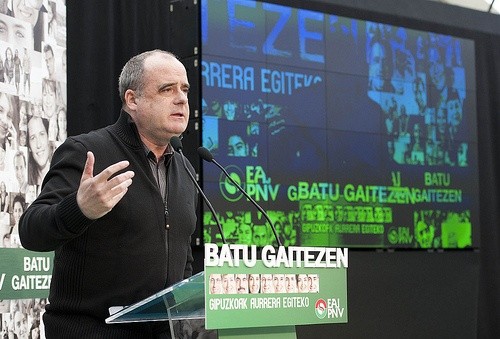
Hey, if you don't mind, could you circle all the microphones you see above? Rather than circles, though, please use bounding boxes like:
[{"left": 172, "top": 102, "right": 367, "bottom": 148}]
[
  {"left": 197, "top": 146, "right": 284, "bottom": 245},
  {"left": 170, "top": 135, "right": 228, "bottom": 244}
]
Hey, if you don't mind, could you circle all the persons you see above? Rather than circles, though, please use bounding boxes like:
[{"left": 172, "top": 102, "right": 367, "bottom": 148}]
[
  {"left": 18, "top": 50, "right": 200, "bottom": 339},
  {"left": 0, "top": 299, "right": 218, "bottom": 339},
  {"left": 1, "top": 0, "right": 67, "bottom": 248},
  {"left": 208, "top": 273, "right": 319, "bottom": 294},
  {"left": 202, "top": 1, "right": 473, "bottom": 249}
]
